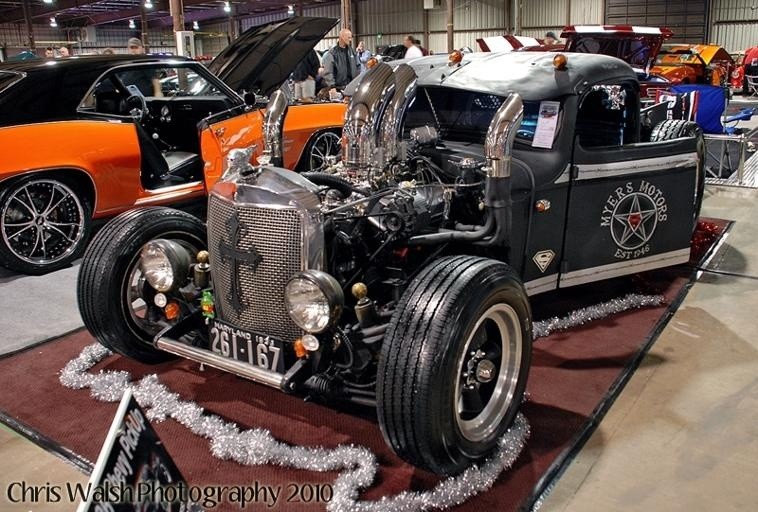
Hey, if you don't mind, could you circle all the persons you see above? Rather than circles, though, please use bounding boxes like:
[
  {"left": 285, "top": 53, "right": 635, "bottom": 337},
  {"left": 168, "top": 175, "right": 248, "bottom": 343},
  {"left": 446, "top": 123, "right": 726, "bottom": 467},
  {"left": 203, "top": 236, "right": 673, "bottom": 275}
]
[
  {"left": 292, "top": 47, "right": 321, "bottom": 101},
  {"left": 44, "top": 47, "right": 54, "bottom": 58},
  {"left": 357, "top": 41, "right": 370, "bottom": 73},
  {"left": 742, "top": 44, "right": 757, "bottom": 99},
  {"left": 544, "top": 31, "right": 557, "bottom": 44},
  {"left": 104, "top": 48, "right": 115, "bottom": 54},
  {"left": 58, "top": 47, "right": 71, "bottom": 58},
  {"left": 122, "top": 37, "right": 164, "bottom": 97},
  {"left": 322, "top": 28, "right": 360, "bottom": 95},
  {"left": 403, "top": 35, "right": 423, "bottom": 58},
  {"left": 412, "top": 39, "right": 428, "bottom": 56}
]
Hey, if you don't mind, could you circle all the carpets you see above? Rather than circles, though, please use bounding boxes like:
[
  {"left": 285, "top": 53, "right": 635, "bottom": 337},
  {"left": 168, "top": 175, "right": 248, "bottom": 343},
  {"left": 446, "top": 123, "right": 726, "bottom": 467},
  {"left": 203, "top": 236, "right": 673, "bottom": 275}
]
[{"left": 0, "top": 212, "right": 736, "bottom": 512}]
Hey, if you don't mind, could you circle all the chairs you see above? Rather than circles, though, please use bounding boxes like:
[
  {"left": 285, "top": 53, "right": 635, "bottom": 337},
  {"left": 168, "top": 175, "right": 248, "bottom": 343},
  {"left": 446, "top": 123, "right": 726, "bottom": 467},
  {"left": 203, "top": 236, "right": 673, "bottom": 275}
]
[{"left": 95, "top": 82, "right": 201, "bottom": 189}]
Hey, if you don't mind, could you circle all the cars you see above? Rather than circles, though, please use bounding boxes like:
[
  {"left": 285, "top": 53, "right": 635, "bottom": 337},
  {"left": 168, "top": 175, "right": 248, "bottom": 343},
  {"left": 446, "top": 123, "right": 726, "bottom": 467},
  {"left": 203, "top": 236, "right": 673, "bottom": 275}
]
[
  {"left": 476, "top": 24, "right": 735, "bottom": 87},
  {"left": 1, "top": 16, "right": 340, "bottom": 274},
  {"left": 76, "top": 51, "right": 705, "bottom": 475}
]
